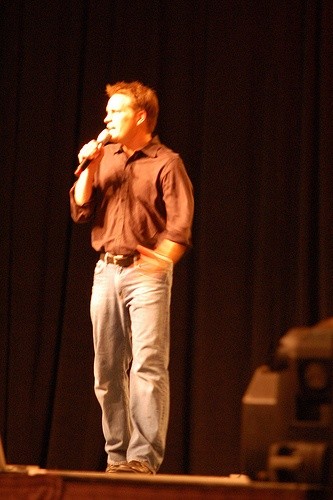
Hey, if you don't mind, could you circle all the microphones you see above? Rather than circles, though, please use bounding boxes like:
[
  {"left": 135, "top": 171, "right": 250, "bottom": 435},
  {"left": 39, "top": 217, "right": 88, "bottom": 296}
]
[{"left": 74, "top": 128, "right": 110, "bottom": 177}]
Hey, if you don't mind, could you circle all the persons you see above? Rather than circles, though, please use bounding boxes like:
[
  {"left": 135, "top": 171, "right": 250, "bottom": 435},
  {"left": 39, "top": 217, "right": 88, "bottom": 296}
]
[{"left": 69, "top": 81, "right": 194, "bottom": 475}]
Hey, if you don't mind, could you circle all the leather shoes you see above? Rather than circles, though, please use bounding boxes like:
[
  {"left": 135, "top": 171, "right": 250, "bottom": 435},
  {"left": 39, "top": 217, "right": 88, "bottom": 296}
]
[
  {"left": 116, "top": 460, "right": 152, "bottom": 474},
  {"left": 106, "top": 465, "right": 119, "bottom": 473}
]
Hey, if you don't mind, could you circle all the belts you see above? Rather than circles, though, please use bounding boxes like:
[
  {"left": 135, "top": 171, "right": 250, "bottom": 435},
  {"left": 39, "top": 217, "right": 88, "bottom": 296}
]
[{"left": 99, "top": 252, "right": 133, "bottom": 269}]
